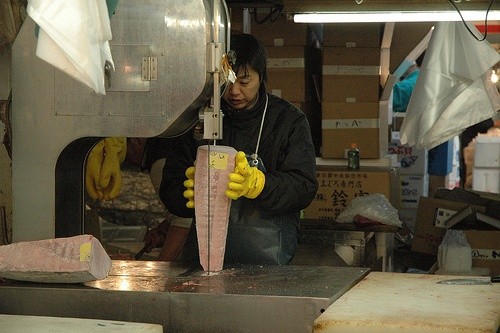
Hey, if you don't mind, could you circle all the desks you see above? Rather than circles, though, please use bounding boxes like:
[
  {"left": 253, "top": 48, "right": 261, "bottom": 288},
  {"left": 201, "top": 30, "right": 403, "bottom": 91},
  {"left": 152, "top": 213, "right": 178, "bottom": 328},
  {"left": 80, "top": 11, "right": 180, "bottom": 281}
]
[
  {"left": 1, "top": 259, "right": 371, "bottom": 333},
  {"left": 312, "top": 271, "right": 500, "bottom": 333}
]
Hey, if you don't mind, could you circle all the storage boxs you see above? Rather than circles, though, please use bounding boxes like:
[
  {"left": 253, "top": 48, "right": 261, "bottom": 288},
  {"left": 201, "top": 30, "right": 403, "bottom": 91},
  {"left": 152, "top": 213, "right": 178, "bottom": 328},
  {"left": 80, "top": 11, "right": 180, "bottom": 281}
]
[
  {"left": 412, "top": 188, "right": 500, "bottom": 273},
  {"left": 303, "top": 166, "right": 401, "bottom": 221}
]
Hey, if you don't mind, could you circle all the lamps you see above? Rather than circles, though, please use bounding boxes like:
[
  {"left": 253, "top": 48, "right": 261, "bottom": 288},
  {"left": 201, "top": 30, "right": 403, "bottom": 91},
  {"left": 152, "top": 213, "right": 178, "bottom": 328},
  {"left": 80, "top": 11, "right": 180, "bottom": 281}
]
[{"left": 284, "top": 4, "right": 500, "bottom": 24}]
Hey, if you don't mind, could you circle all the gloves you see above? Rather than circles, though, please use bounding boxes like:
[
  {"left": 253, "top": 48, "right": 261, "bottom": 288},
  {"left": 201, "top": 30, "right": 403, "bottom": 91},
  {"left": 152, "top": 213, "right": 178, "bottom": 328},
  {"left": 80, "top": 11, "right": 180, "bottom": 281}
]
[
  {"left": 183, "top": 160, "right": 196, "bottom": 209},
  {"left": 86, "top": 139, "right": 104, "bottom": 200},
  {"left": 100, "top": 137, "right": 128, "bottom": 201},
  {"left": 225, "top": 150, "right": 266, "bottom": 201}
]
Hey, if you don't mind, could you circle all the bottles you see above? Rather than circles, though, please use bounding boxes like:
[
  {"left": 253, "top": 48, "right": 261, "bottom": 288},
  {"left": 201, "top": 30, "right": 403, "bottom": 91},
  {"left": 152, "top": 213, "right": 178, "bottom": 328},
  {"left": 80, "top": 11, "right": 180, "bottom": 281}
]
[{"left": 347, "top": 144, "right": 360, "bottom": 169}]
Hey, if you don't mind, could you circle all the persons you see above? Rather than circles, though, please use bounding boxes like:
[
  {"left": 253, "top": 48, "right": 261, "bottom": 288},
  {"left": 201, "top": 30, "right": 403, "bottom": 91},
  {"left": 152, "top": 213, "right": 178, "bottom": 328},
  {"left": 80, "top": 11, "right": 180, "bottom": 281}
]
[
  {"left": 158, "top": 33, "right": 319, "bottom": 266},
  {"left": 124, "top": 136, "right": 193, "bottom": 263},
  {"left": 393, "top": 48, "right": 427, "bottom": 111},
  {"left": 83, "top": 67, "right": 146, "bottom": 241}
]
